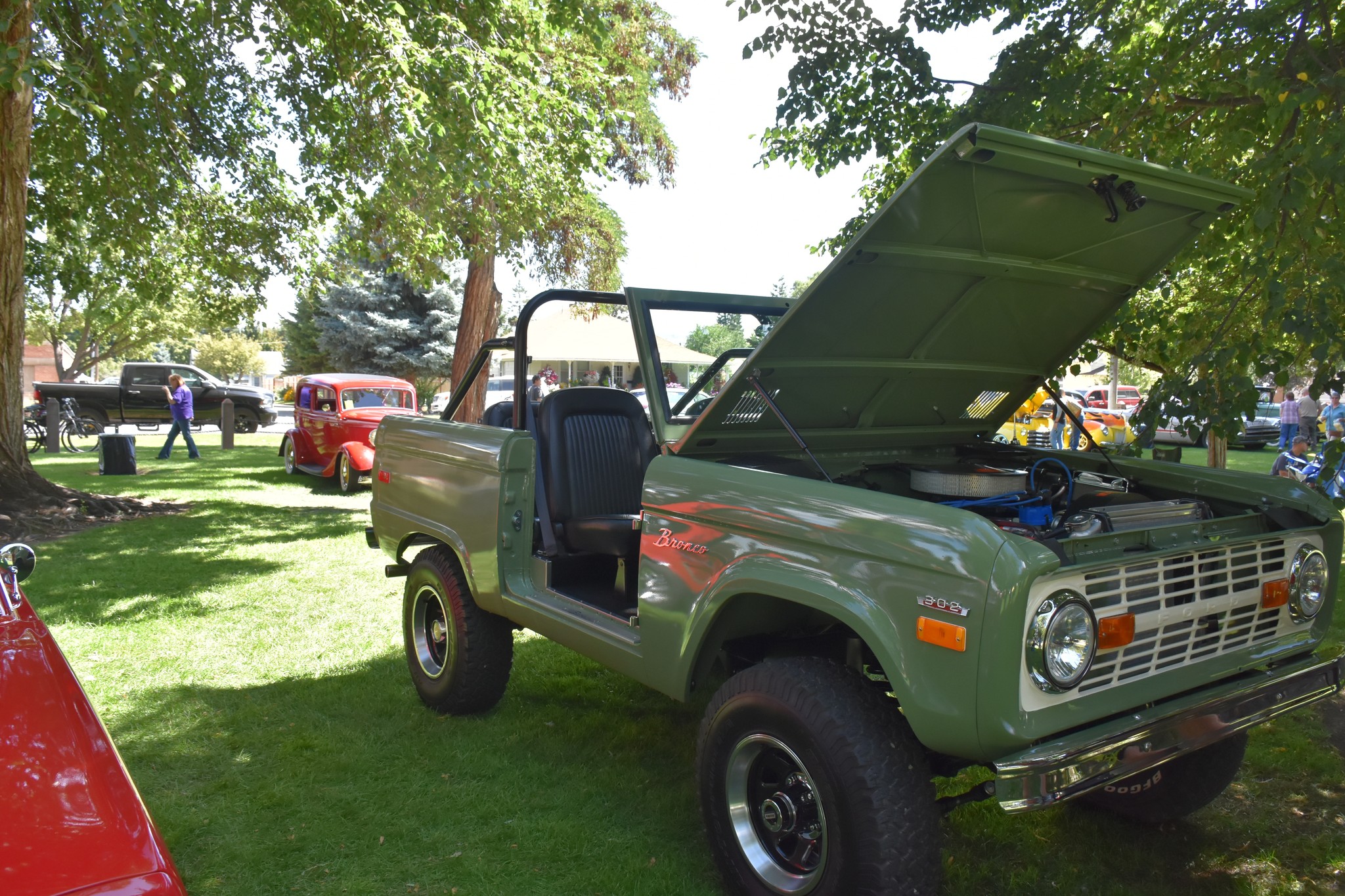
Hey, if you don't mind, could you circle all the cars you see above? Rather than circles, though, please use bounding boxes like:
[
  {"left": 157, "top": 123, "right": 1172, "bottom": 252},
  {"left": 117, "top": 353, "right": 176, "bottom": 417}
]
[
  {"left": 0, "top": 541, "right": 193, "bottom": 895},
  {"left": 967, "top": 383, "right": 1281, "bottom": 454},
  {"left": 629, "top": 387, "right": 780, "bottom": 423},
  {"left": 278, "top": 373, "right": 424, "bottom": 493},
  {"left": 359, "top": 120, "right": 1345, "bottom": 895}
]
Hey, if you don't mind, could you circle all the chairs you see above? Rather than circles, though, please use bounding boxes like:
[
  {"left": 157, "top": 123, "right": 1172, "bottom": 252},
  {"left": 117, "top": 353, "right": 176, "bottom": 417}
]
[
  {"left": 1282, "top": 437, "right": 1345, "bottom": 498},
  {"left": 1152, "top": 447, "right": 1182, "bottom": 462},
  {"left": 483, "top": 386, "right": 660, "bottom": 558}
]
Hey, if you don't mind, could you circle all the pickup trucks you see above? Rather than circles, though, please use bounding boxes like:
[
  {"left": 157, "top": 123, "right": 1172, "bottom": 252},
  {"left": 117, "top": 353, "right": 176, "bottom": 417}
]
[{"left": 32, "top": 362, "right": 279, "bottom": 435}]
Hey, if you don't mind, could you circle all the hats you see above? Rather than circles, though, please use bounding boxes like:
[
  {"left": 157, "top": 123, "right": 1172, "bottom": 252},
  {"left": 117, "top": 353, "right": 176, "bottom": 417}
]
[
  {"left": 532, "top": 375, "right": 541, "bottom": 381},
  {"left": 1292, "top": 436, "right": 1314, "bottom": 444}
]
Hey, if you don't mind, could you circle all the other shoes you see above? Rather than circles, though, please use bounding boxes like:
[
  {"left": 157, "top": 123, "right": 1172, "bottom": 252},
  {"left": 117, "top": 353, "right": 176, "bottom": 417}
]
[{"left": 1277, "top": 447, "right": 1283, "bottom": 453}]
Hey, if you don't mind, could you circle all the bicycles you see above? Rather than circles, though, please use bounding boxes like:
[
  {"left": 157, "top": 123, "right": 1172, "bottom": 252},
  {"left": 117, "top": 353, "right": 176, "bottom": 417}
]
[{"left": 23, "top": 397, "right": 105, "bottom": 454}]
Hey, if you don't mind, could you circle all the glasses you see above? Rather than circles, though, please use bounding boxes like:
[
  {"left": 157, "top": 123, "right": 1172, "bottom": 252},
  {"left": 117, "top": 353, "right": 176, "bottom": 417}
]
[
  {"left": 1332, "top": 397, "right": 1338, "bottom": 399},
  {"left": 1307, "top": 444, "right": 1311, "bottom": 446}
]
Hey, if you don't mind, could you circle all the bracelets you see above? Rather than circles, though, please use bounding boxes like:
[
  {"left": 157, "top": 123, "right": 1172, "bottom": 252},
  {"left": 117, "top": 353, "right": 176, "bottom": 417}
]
[{"left": 1054, "top": 420, "right": 1058, "bottom": 423}]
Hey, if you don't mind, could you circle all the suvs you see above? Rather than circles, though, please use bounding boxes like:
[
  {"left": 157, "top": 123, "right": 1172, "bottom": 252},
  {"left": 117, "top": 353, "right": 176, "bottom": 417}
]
[{"left": 430, "top": 373, "right": 549, "bottom": 416}]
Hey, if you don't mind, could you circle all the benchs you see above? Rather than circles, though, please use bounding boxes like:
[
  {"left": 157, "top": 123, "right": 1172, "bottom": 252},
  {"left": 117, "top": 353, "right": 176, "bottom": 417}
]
[{"left": 317, "top": 399, "right": 354, "bottom": 411}]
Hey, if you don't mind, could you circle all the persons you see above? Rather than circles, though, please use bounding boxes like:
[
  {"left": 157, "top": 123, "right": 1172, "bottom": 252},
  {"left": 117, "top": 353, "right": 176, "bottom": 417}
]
[
  {"left": 155, "top": 374, "right": 202, "bottom": 461},
  {"left": 1063, "top": 394, "right": 1085, "bottom": 450},
  {"left": 1270, "top": 435, "right": 1316, "bottom": 489},
  {"left": 1320, "top": 392, "right": 1345, "bottom": 446},
  {"left": 1277, "top": 392, "right": 1301, "bottom": 453},
  {"left": 1296, "top": 387, "right": 1323, "bottom": 451},
  {"left": 529, "top": 374, "right": 544, "bottom": 401},
  {"left": 1048, "top": 389, "right": 1067, "bottom": 450}
]
